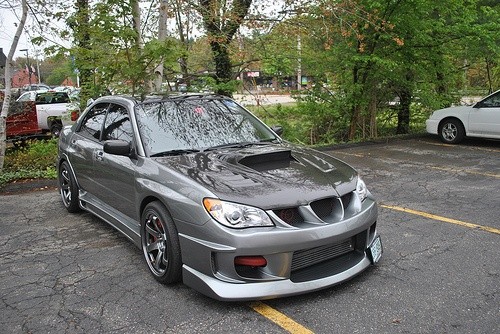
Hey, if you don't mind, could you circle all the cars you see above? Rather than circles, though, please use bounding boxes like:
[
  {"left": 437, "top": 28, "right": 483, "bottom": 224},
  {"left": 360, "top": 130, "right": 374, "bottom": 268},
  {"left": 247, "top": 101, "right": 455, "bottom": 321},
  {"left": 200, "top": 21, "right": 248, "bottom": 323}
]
[
  {"left": 58, "top": 92, "right": 382, "bottom": 301},
  {"left": 15, "top": 90, "right": 79, "bottom": 133},
  {"left": 23, "top": 83, "right": 81, "bottom": 99},
  {"left": 425, "top": 90, "right": 500, "bottom": 143}
]
